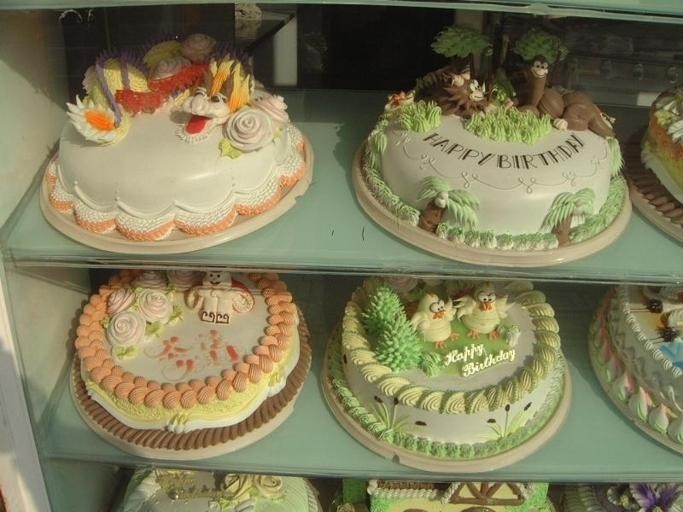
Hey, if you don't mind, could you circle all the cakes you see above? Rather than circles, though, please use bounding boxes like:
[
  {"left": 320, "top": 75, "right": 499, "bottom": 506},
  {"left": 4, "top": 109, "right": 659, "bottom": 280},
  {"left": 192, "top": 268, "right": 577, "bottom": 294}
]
[
  {"left": 562, "top": 483, "right": 683, "bottom": 511},
  {"left": 639, "top": 65, "right": 682, "bottom": 209},
  {"left": 46, "top": 32, "right": 307, "bottom": 241},
  {"left": 589, "top": 284, "right": 682, "bottom": 452},
  {"left": 116, "top": 464, "right": 323, "bottom": 511},
  {"left": 73, "top": 269, "right": 312, "bottom": 450},
  {"left": 327, "top": 275, "right": 565, "bottom": 457},
  {"left": 360, "top": 24, "right": 627, "bottom": 253},
  {"left": 365, "top": 480, "right": 555, "bottom": 512}
]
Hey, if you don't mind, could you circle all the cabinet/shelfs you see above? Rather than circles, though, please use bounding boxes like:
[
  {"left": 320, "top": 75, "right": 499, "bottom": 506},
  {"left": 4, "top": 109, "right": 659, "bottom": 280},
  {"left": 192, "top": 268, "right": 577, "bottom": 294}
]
[{"left": 0, "top": 0, "right": 683, "bottom": 512}]
